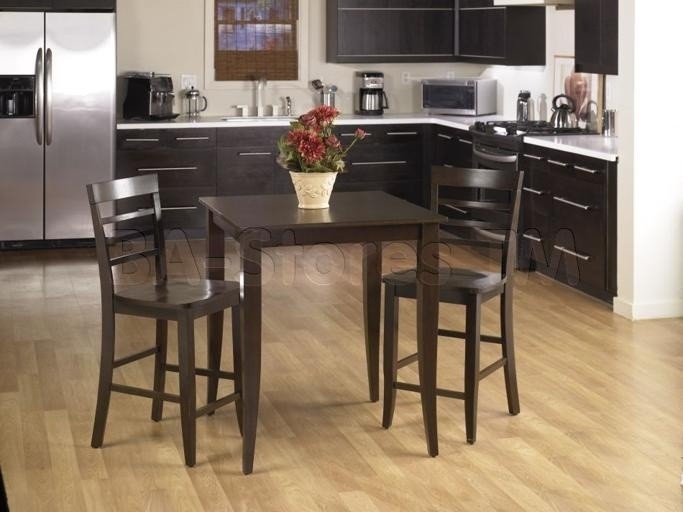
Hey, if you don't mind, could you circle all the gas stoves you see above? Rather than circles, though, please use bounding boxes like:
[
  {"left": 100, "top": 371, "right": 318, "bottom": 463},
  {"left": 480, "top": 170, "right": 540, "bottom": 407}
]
[{"left": 486, "top": 120, "right": 600, "bottom": 136}]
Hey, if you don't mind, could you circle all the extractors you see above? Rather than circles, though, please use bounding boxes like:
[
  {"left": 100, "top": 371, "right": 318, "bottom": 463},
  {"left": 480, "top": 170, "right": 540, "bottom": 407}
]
[{"left": 490, "top": 0, "right": 576, "bottom": 7}]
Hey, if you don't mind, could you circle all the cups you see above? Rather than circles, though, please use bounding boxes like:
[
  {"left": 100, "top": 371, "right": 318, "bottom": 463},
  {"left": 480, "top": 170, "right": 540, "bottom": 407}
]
[
  {"left": 185, "top": 86, "right": 207, "bottom": 117},
  {"left": 602, "top": 110, "right": 615, "bottom": 137}
]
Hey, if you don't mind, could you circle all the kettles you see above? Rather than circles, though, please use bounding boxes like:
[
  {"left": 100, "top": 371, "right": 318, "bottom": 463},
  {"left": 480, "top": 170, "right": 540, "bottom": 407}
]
[{"left": 549, "top": 94, "right": 580, "bottom": 130}]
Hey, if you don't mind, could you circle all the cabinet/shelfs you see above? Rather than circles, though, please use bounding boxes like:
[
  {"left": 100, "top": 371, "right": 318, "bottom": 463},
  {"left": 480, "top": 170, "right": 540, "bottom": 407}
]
[
  {"left": 325, "top": 0, "right": 457, "bottom": 62},
  {"left": 217, "top": 129, "right": 298, "bottom": 196},
  {"left": 428, "top": 125, "right": 472, "bottom": 240},
  {"left": 116, "top": 129, "right": 216, "bottom": 240},
  {"left": 457, "top": 0, "right": 545, "bottom": 66},
  {"left": 517, "top": 135, "right": 619, "bottom": 308},
  {"left": 329, "top": 126, "right": 429, "bottom": 209}
]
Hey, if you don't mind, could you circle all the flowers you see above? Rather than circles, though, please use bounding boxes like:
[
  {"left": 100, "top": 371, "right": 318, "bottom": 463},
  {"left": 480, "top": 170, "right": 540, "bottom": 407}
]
[{"left": 273, "top": 105, "right": 368, "bottom": 172}]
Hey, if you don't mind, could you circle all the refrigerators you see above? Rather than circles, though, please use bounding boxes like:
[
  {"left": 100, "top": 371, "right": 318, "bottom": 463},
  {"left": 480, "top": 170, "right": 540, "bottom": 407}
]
[{"left": 0, "top": 1, "right": 116, "bottom": 251}]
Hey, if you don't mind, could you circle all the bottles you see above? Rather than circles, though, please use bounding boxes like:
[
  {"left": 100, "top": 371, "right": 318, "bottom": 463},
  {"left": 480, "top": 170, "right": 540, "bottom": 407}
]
[{"left": 517, "top": 89, "right": 529, "bottom": 124}]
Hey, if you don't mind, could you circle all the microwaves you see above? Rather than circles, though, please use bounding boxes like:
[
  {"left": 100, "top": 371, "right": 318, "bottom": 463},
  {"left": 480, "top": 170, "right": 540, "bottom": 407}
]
[{"left": 419, "top": 77, "right": 498, "bottom": 118}]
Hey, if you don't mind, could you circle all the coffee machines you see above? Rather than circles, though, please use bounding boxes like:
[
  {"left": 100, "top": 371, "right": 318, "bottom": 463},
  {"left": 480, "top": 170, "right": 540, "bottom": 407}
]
[{"left": 353, "top": 70, "right": 389, "bottom": 116}]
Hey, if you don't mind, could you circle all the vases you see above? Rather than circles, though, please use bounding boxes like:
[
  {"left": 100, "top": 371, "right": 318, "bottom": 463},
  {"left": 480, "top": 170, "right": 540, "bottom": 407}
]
[{"left": 290, "top": 170, "right": 339, "bottom": 208}]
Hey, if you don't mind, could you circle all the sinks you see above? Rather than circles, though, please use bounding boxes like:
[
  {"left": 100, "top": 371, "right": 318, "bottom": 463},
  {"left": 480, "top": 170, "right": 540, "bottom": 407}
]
[{"left": 221, "top": 116, "right": 298, "bottom": 121}]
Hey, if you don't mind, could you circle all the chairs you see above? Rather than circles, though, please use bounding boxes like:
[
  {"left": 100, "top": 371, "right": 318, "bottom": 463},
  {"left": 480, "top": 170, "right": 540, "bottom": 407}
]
[
  {"left": 380, "top": 166, "right": 528, "bottom": 445},
  {"left": 86, "top": 173, "right": 250, "bottom": 467}
]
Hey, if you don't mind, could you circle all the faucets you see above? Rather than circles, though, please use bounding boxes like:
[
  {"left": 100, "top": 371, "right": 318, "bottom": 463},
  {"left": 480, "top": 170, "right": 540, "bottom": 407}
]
[{"left": 255, "top": 76, "right": 268, "bottom": 118}]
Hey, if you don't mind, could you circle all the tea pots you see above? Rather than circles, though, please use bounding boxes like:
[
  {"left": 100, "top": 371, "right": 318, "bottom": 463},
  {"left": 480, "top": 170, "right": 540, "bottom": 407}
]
[{"left": 5, "top": 85, "right": 19, "bottom": 117}]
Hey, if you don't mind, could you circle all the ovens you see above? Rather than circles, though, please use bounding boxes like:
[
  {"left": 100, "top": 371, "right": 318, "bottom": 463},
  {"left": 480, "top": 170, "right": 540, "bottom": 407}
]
[{"left": 472, "top": 144, "right": 524, "bottom": 233}]
